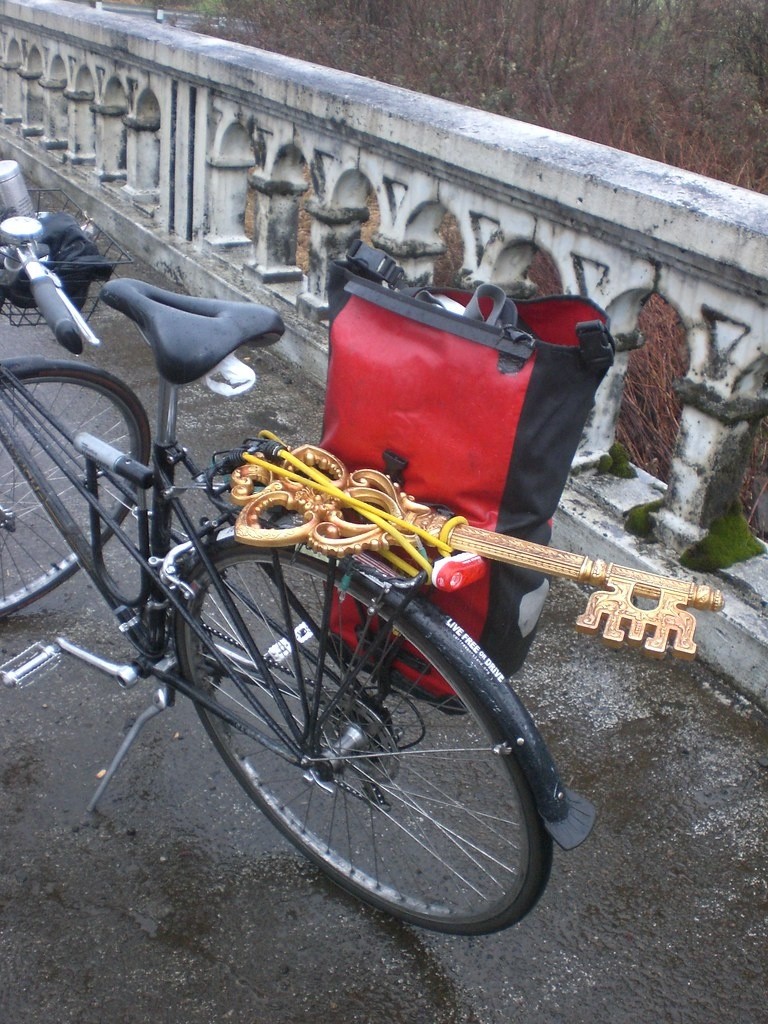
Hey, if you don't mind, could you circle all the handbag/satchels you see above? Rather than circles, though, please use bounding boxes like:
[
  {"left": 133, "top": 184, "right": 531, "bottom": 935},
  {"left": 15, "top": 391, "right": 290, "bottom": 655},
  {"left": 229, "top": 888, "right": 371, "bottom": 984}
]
[{"left": 319, "top": 242, "right": 617, "bottom": 714}]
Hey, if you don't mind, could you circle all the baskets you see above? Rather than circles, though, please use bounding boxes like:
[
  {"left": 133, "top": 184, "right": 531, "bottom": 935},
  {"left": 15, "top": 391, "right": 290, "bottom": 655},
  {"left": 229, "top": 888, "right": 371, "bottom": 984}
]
[{"left": 0, "top": 187, "right": 134, "bottom": 326}]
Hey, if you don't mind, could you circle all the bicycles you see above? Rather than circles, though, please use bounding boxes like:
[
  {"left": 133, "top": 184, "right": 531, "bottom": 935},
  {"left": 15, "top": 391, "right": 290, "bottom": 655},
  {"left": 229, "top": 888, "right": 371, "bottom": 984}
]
[{"left": 1, "top": 210, "right": 600, "bottom": 937}]
